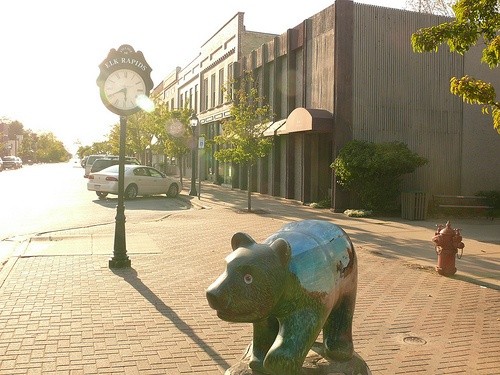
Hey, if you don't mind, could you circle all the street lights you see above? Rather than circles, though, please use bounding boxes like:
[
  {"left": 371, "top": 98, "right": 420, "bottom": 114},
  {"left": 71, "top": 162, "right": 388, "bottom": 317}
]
[{"left": 189, "top": 113, "right": 199, "bottom": 197}]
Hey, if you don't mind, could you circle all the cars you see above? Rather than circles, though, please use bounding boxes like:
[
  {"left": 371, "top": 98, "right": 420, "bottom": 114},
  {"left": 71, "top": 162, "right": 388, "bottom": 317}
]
[
  {"left": 81, "top": 154, "right": 141, "bottom": 179},
  {"left": 88, "top": 165, "right": 182, "bottom": 199},
  {"left": 0, "top": 157, "right": 23, "bottom": 170}
]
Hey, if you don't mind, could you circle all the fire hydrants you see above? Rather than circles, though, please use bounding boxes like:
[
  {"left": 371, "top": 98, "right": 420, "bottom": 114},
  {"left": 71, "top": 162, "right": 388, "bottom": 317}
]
[{"left": 432, "top": 221, "right": 465, "bottom": 279}]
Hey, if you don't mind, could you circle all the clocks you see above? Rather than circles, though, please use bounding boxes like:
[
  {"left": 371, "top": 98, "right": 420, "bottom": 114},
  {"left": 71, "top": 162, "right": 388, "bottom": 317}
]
[{"left": 100, "top": 63, "right": 150, "bottom": 117}]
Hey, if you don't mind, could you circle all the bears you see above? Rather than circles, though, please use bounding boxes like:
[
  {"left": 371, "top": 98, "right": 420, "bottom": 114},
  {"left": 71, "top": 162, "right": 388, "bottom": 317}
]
[{"left": 205, "top": 219, "right": 358, "bottom": 375}]
